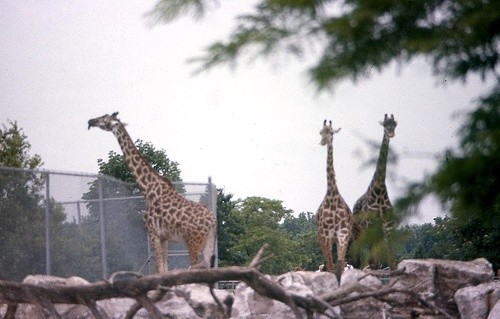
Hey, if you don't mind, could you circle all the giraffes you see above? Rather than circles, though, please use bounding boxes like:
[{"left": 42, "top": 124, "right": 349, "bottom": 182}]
[
  {"left": 87, "top": 112, "right": 218, "bottom": 274},
  {"left": 315, "top": 119, "right": 352, "bottom": 272},
  {"left": 352, "top": 114, "right": 398, "bottom": 273}
]
